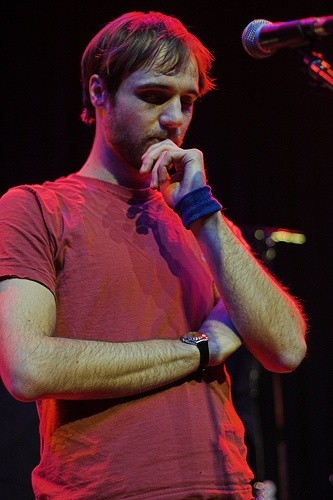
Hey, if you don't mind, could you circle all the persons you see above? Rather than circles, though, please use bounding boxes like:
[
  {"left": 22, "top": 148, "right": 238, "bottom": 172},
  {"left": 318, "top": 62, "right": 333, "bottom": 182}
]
[{"left": 0, "top": 12, "right": 306, "bottom": 500}]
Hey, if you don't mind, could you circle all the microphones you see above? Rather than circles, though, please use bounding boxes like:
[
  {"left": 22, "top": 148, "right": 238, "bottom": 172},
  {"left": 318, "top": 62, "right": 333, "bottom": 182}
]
[{"left": 241, "top": 14, "right": 333, "bottom": 59}]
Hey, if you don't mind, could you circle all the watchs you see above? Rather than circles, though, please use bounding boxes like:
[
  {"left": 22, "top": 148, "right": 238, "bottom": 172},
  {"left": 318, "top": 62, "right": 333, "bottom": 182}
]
[{"left": 181, "top": 332, "right": 209, "bottom": 373}]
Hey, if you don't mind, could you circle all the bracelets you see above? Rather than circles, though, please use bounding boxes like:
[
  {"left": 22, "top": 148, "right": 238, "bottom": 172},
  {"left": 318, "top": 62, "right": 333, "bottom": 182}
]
[{"left": 173, "top": 186, "right": 220, "bottom": 230}]
